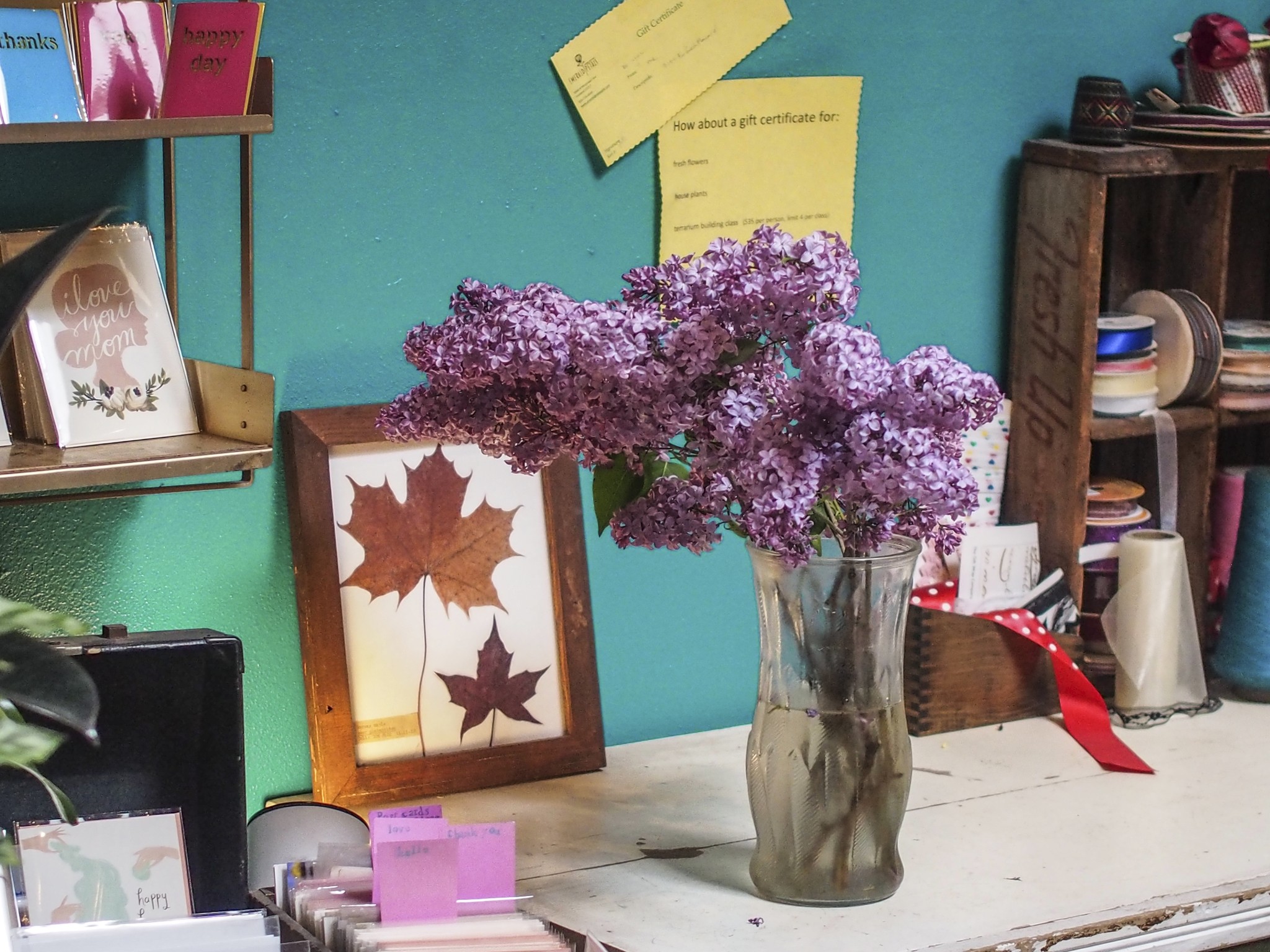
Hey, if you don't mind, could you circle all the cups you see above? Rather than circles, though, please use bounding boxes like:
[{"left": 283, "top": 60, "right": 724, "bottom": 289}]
[{"left": 1071, "top": 75, "right": 1130, "bottom": 146}]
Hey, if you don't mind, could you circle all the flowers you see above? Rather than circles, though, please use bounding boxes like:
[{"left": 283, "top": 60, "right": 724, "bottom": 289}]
[{"left": 368, "top": 215, "right": 1000, "bottom": 571}]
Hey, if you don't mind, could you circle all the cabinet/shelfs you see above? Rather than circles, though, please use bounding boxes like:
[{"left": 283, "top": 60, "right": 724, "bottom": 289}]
[
  {"left": 0, "top": 57, "right": 277, "bottom": 507},
  {"left": 996, "top": 138, "right": 1270, "bottom": 695}
]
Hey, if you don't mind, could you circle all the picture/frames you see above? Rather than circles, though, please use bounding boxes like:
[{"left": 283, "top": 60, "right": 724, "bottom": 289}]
[{"left": 279, "top": 404, "right": 609, "bottom": 811}]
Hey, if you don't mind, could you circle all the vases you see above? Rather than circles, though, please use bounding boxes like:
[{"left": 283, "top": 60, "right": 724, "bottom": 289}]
[{"left": 739, "top": 528, "right": 922, "bottom": 901}]
